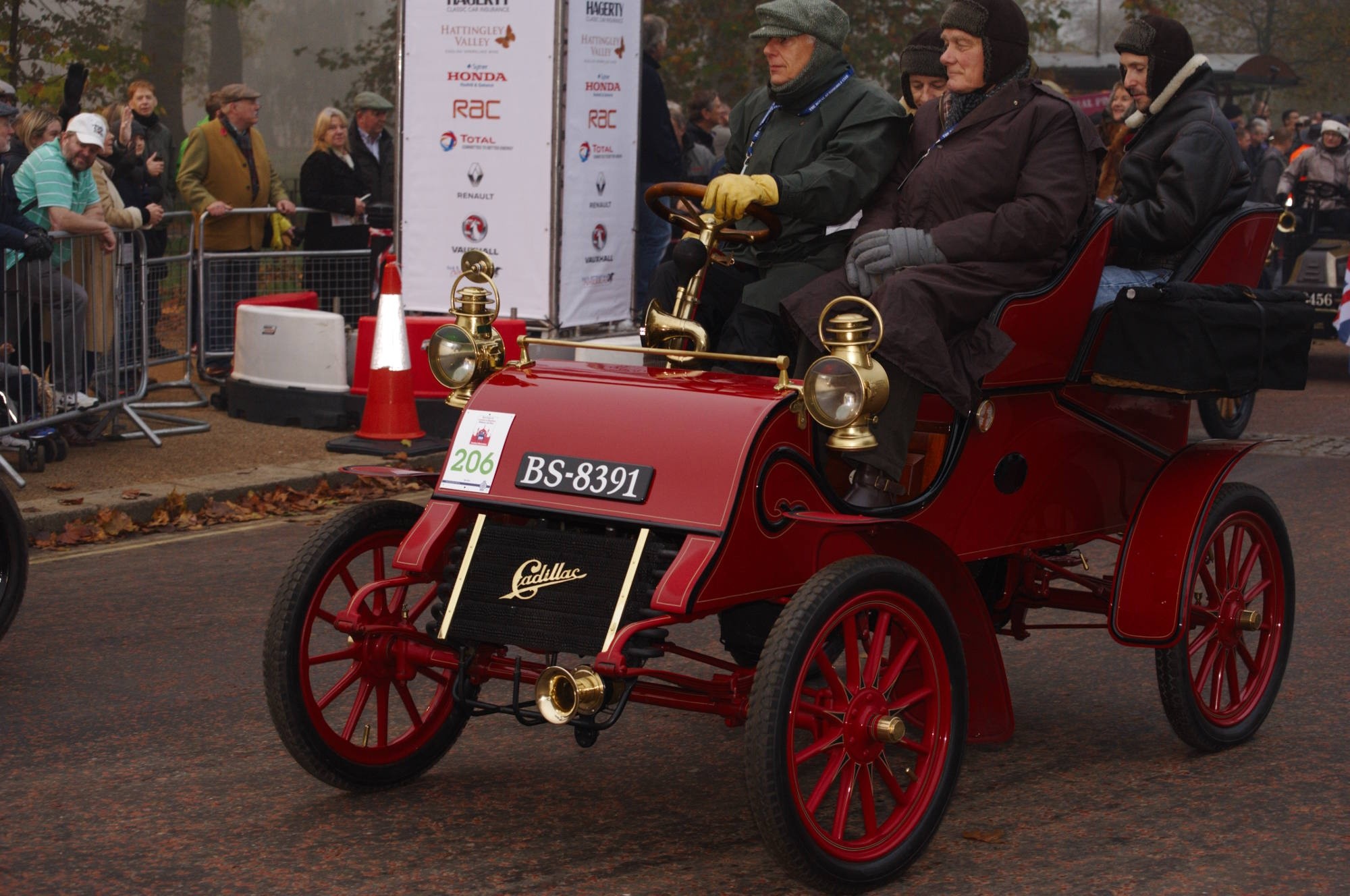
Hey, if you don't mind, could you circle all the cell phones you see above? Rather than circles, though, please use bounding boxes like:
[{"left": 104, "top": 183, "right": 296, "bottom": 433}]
[
  {"left": 360, "top": 193, "right": 372, "bottom": 203},
  {"left": 153, "top": 156, "right": 163, "bottom": 161}
]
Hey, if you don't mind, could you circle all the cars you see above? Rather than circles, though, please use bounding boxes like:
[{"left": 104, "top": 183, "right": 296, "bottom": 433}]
[{"left": 261, "top": 181, "right": 1296, "bottom": 896}]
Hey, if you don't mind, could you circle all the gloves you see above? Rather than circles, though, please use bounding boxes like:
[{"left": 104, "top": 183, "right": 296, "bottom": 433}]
[
  {"left": 847, "top": 244, "right": 897, "bottom": 296},
  {"left": 701, "top": 172, "right": 780, "bottom": 221},
  {"left": 852, "top": 227, "right": 947, "bottom": 273}
]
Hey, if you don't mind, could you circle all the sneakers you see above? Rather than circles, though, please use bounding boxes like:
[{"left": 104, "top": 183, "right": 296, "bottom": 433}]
[{"left": 52, "top": 391, "right": 99, "bottom": 410}]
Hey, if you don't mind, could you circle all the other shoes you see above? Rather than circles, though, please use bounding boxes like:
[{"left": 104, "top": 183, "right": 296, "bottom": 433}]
[{"left": 198, "top": 366, "right": 225, "bottom": 380}]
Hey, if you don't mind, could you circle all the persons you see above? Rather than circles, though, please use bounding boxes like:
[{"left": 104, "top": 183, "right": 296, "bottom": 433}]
[
  {"left": 0, "top": 78, "right": 395, "bottom": 452},
  {"left": 653, "top": 0, "right": 1253, "bottom": 508},
  {"left": 634, "top": 14, "right": 731, "bottom": 326},
  {"left": 1222, "top": 100, "right": 1350, "bottom": 291}
]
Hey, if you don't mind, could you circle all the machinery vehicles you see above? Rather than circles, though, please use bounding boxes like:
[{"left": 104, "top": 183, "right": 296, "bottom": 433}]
[{"left": 1277, "top": 178, "right": 1350, "bottom": 342}]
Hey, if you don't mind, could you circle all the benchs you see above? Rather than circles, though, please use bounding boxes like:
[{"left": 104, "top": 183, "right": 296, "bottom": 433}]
[
  {"left": 1167, "top": 201, "right": 1286, "bottom": 286},
  {"left": 977, "top": 198, "right": 1119, "bottom": 390}
]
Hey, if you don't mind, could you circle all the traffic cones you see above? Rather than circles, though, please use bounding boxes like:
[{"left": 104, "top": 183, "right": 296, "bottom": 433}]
[{"left": 324, "top": 253, "right": 452, "bottom": 457}]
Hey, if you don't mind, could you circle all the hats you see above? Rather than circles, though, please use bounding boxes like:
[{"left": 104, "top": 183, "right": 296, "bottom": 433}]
[
  {"left": 1320, "top": 112, "right": 1350, "bottom": 143},
  {"left": 940, "top": 1, "right": 1030, "bottom": 87},
  {"left": 898, "top": 30, "right": 948, "bottom": 107},
  {"left": 1113, "top": 14, "right": 1191, "bottom": 104},
  {"left": 220, "top": 83, "right": 262, "bottom": 103},
  {"left": 749, "top": 0, "right": 850, "bottom": 93},
  {"left": 353, "top": 91, "right": 393, "bottom": 111},
  {"left": 67, "top": 113, "right": 109, "bottom": 153}
]
